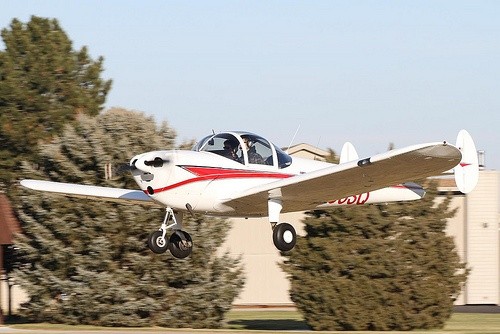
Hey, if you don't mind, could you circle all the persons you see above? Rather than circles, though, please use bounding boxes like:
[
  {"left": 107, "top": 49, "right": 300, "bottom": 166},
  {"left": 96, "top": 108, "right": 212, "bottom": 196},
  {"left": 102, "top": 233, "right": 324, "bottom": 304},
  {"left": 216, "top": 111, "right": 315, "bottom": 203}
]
[
  {"left": 223, "top": 139, "right": 239, "bottom": 162},
  {"left": 236, "top": 135, "right": 266, "bottom": 166}
]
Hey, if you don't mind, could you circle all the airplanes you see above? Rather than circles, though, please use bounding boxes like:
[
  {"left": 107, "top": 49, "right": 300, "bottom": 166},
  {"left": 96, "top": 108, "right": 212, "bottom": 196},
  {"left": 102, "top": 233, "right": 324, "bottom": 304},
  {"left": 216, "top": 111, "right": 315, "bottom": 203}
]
[{"left": 19, "top": 120, "right": 462, "bottom": 261}]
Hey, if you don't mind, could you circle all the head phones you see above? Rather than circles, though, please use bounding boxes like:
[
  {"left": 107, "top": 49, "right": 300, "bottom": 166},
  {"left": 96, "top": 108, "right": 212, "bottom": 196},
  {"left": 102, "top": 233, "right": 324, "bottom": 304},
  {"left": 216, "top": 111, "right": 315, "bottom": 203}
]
[{"left": 245, "top": 136, "right": 253, "bottom": 148}]
